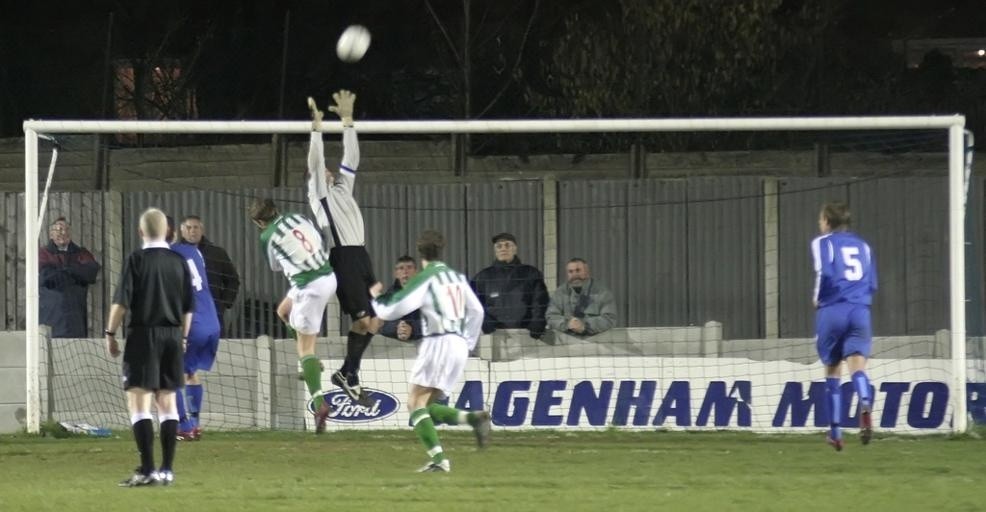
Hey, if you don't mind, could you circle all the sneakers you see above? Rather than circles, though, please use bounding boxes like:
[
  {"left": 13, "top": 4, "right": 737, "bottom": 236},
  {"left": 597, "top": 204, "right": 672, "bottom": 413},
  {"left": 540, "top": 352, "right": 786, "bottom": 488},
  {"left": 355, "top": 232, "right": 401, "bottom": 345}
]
[
  {"left": 176, "top": 426, "right": 202, "bottom": 443},
  {"left": 329, "top": 370, "right": 370, "bottom": 409},
  {"left": 861, "top": 405, "right": 875, "bottom": 445},
  {"left": 307, "top": 401, "right": 332, "bottom": 434},
  {"left": 824, "top": 432, "right": 845, "bottom": 454},
  {"left": 112, "top": 472, "right": 177, "bottom": 486},
  {"left": 415, "top": 458, "right": 451, "bottom": 475},
  {"left": 469, "top": 408, "right": 491, "bottom": 452}
]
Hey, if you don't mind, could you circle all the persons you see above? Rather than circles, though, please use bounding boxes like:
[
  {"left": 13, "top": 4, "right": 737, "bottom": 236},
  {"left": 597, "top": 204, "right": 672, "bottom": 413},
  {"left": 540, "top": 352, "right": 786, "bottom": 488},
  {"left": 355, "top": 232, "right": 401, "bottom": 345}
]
[
  {"left": 181, "top": 217, "right": 241, "bottom": 338},
  {"left": 39, "top": 217, "right": 100, "bottom": 340},
  {"left": 248, "top": 196, "right": 338, "bottom": 435},
  {"left": 369, "top": 230, "right": 493, "bottom": 475},
  {"left": 808, "top": 200, "right": 879, "bottom": 450},
  {"left": 544, "top": 258, "right": 620, "bottom": 338},
  {"left": 467, "top": 232, "right": 550, "bottom": 342},
  {"left": 305, "top": 89, "right": 382, "bottom": 406},
  {"left": 164, "top": 218, "right": 222, "bottom": 442},
  {"left": 105, "top": 208, "right": 197, "bottom": 489},
  {"left": 377, "top": 258, "right": 422, "bottom": 340}
]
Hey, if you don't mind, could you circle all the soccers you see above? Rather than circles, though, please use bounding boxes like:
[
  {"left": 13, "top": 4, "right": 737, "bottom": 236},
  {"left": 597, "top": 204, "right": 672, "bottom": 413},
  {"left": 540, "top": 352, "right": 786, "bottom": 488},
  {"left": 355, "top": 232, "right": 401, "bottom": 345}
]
[{"left": 336, "top": 25, "right": 370, "bottom": 62}]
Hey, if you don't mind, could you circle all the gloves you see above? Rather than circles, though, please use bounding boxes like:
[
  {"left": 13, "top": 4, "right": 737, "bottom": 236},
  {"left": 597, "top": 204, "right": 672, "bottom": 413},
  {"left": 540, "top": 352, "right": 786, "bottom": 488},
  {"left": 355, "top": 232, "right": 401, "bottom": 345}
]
[
  {"left": 528, "top": 319, "right": 546, "bottom": 340},
  {"left": 481, "top": 315, "right": 495, "bottom": 335},
  {"left": 396, "top": 320, "right": 413, "bottom": 342}
]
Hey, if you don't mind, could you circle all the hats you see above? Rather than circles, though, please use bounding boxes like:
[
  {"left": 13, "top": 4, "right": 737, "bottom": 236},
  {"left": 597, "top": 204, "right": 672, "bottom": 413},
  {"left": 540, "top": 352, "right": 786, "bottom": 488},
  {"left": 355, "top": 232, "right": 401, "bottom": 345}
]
[{"left": 491, "top": 229, "right": 518, "bottom": 245}]
